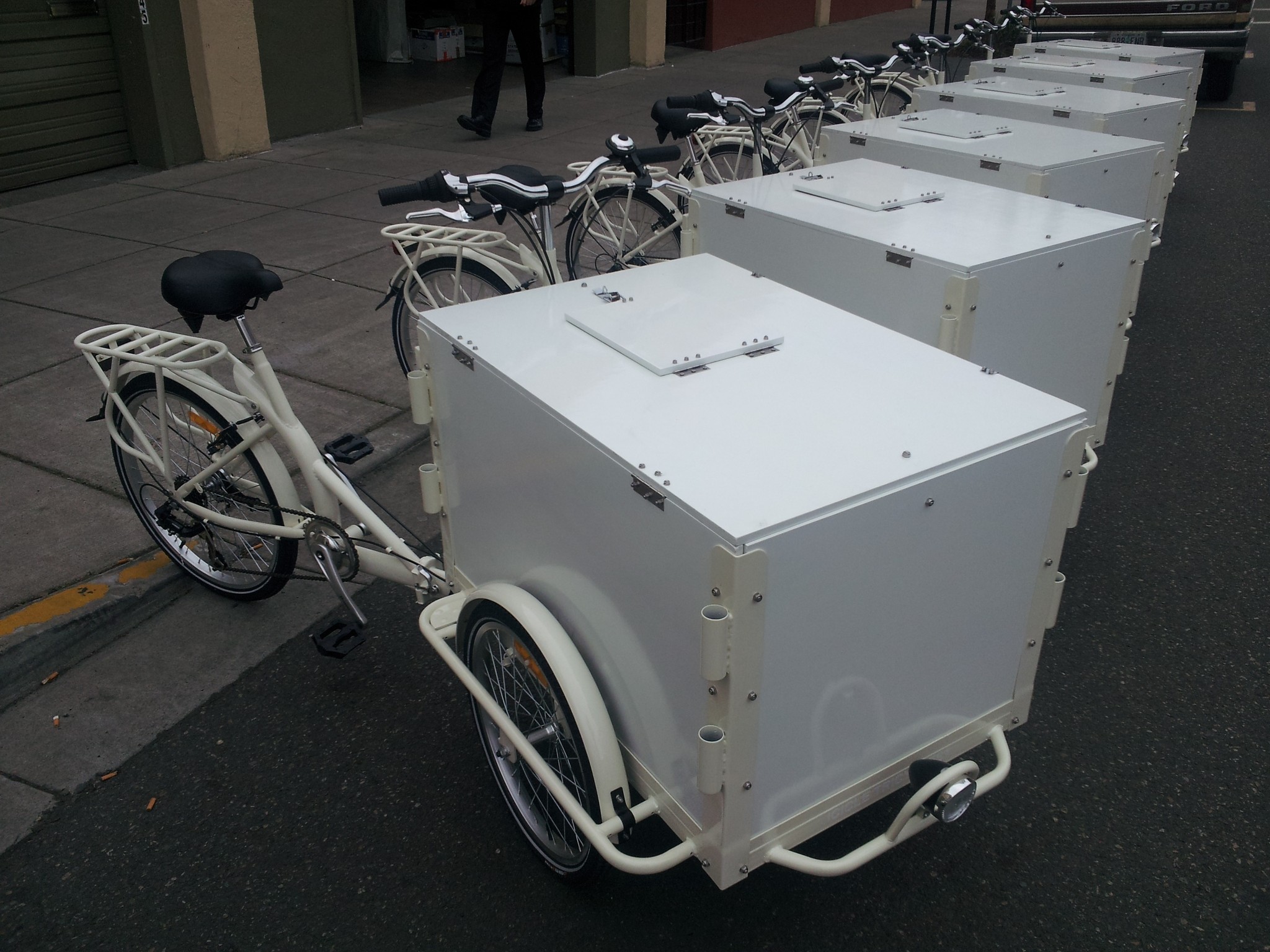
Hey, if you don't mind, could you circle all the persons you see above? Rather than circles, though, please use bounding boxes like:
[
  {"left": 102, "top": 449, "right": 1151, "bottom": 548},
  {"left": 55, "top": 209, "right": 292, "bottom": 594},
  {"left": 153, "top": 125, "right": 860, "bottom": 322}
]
[{"left": 456, "top": 0, "right": 548, "bottom": 137}]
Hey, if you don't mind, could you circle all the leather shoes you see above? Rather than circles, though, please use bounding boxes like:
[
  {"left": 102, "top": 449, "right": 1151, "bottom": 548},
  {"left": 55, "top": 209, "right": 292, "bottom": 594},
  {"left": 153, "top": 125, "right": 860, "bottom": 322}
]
[
  {"left": 526, "top": 117, "right": 543, "bottom": 131},
  {"left": 457, "top": 113, "right": 491, "bottom": 138}
]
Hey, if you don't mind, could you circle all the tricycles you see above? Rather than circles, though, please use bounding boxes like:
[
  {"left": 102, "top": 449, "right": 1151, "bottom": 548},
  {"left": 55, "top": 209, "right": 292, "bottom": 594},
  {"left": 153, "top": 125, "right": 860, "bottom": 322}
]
[{"left": 74, "top": 0, "right": 1206, "bottom": 900}]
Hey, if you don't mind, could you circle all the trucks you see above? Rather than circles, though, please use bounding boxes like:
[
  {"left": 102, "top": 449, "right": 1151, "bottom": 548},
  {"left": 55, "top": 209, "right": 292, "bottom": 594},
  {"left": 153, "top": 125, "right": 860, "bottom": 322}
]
[{"left": 1019, "top": 0, "right": 1255, "bottom": 93}]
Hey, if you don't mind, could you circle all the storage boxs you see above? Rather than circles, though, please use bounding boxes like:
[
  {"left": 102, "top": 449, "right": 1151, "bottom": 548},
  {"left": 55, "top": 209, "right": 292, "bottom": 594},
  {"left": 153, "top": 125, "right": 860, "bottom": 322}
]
[
  {"left": 406, "top": 250, "right": 1099, "bottom": 896},
  {"left": 1012, "top": 37, "right": 1206, "bottom": 138},
  {"left": 906, "top": 76, "right": 1186, "bottom": 194},
  {"left": 965, "top": 54, "right": 1194, "bottom": 154},
  {"left": 816, "top": 107, "right": 1166, "bottom": 242},
  {"left": 680, "top": 156, "right": 1163, "bottom": 462}
]
